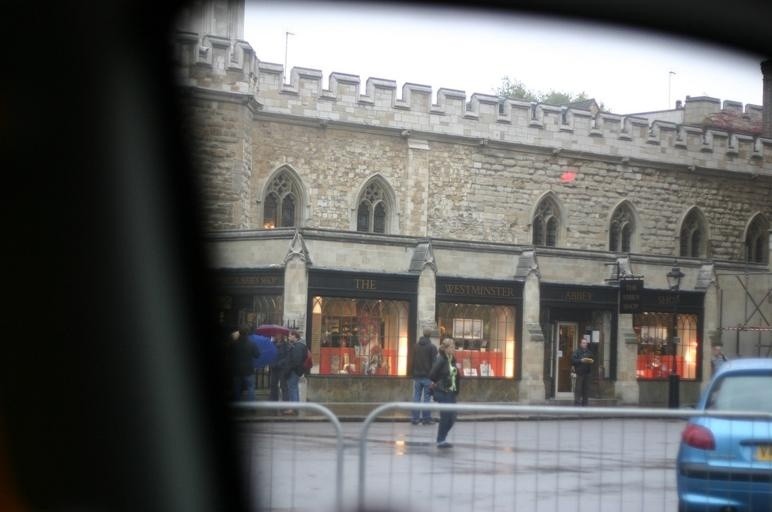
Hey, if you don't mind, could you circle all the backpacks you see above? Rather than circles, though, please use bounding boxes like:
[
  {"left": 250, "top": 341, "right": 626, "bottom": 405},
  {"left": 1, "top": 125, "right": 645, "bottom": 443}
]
[{"left": 293, "top": 342, "right": 314, "bottom": 370}]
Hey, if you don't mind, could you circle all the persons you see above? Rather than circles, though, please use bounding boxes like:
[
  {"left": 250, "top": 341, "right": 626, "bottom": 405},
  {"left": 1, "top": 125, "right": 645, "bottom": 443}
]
[
  {"left": 231, "top": 324, "right": 258, "bottom": 417},
  {"left": 282, "top": 331, "right": 307, "bottom": 416},
  {"left": 413, "top": 329, "right": 438, "bottom": 426},
  {"left": 570, "top": 337, "right": 596, "bottom": 406},
  {"left": 270, "top": 333, "right": 289, "bottom": 415},
  {"left": 710, "top": 345, "right": 728, "bottom": 376},
  {"left": 428, "top": 336, "right": 459, "bottom": 448}
]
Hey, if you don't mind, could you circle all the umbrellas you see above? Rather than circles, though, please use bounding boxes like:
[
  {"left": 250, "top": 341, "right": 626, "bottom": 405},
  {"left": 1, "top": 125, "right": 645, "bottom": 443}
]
[
  {"left": 250, "top": 334, "right": 280, "bottom": 369},
  {"left": 254, "top": 323, "right": 290, "bottom": 337}
]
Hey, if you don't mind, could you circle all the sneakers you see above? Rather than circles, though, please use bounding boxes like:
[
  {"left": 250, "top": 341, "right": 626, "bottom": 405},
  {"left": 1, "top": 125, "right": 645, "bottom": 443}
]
[
  {"left": 573, "top": 401, "right": 591, "bottom": 407},
  {"left": 281, "top": 409, "right": 300, "bottom": 416},
  {"left": 411, "top": 417, "right": 454, "bottom": 450}
]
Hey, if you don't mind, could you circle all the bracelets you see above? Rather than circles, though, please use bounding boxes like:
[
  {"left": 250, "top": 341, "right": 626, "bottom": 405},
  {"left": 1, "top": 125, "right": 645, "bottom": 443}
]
[{"left": 431, "top": 381, "right": 435, "bottom": 384}]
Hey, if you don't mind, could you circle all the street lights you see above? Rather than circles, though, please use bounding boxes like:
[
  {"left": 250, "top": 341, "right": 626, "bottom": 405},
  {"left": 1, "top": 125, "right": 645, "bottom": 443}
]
[{"left": 666, "top": 257, "right": 685, "bottom": 408}]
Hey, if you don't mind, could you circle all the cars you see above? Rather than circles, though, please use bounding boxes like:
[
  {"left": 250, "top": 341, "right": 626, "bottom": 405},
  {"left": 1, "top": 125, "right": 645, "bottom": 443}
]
[{"left": 676, "top": 357, "right": 772, "bottom": 512}]
